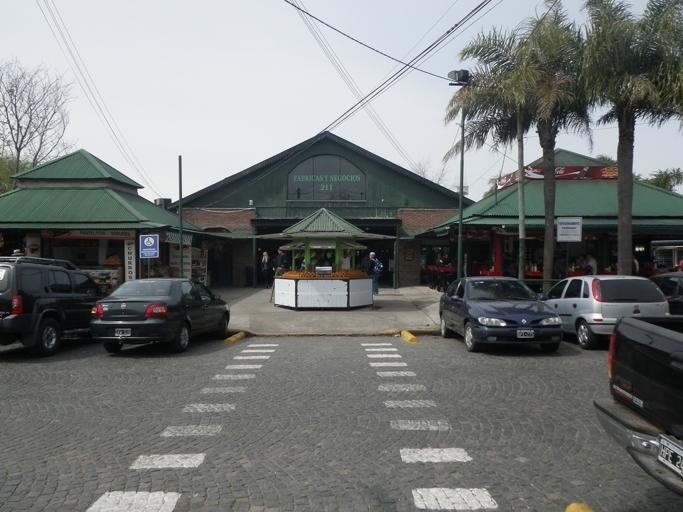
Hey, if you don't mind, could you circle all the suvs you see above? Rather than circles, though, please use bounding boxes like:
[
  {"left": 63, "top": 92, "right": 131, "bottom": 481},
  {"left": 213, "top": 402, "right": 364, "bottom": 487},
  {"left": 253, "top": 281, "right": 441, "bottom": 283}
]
[
  {"left": 0, "top": 256, "right": 112, "bottom": 357},
  {"left": 138, "top": 308, "right": 141, "bottom": 311}
]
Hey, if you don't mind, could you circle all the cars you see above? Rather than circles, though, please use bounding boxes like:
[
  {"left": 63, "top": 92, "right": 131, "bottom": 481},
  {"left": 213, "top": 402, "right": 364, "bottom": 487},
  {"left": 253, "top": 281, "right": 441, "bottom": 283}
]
[
  {"left": 440, "top": 272, "right": 683, "bottom": 352},
  {"left": 90, "top": 277, "right": 230, "bottom": 353}
]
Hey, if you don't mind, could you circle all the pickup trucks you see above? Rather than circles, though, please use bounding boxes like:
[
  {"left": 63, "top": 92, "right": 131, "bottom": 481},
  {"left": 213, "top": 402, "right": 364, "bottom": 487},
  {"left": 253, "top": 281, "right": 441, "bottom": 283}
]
[{"left": 593, "top": 315, "right": 683, "bottom": 498}]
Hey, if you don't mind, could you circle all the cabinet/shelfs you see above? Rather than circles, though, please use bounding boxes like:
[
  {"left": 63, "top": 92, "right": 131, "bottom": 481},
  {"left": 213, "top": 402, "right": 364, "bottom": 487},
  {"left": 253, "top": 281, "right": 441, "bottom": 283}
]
[{"left": 191, "top": 248, "right": 208, "bottom": 287}]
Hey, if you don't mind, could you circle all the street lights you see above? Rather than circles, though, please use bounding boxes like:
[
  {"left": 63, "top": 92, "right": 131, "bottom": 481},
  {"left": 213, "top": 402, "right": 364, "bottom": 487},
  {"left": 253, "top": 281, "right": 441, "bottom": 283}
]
[{"left": 449, "top": 69, "right": 469, "bottom": 277}]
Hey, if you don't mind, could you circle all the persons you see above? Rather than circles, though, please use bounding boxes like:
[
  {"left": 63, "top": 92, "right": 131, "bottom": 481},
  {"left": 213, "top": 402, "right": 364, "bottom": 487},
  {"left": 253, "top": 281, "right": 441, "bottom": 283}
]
[
  {"left": 271, "top": 248, "right": 284, "bottom": 288},
  {"left": 441, "top": 253, "right": 452, "bottom": 268},
  {"left": 259, "top": 251, "right": 273, "bottom": 289},
  {"left": 368, "top": 251, "right": 378, "bottom": 296},
  {"left": 576, "top": 252, "right": 598, "bottom": 275}
]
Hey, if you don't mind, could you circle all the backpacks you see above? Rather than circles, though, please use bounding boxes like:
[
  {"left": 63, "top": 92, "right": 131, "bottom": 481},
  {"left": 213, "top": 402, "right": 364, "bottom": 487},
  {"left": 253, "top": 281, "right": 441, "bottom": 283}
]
[{"left": 369, "top": 257, "right": 384, "bottom": 275}]
